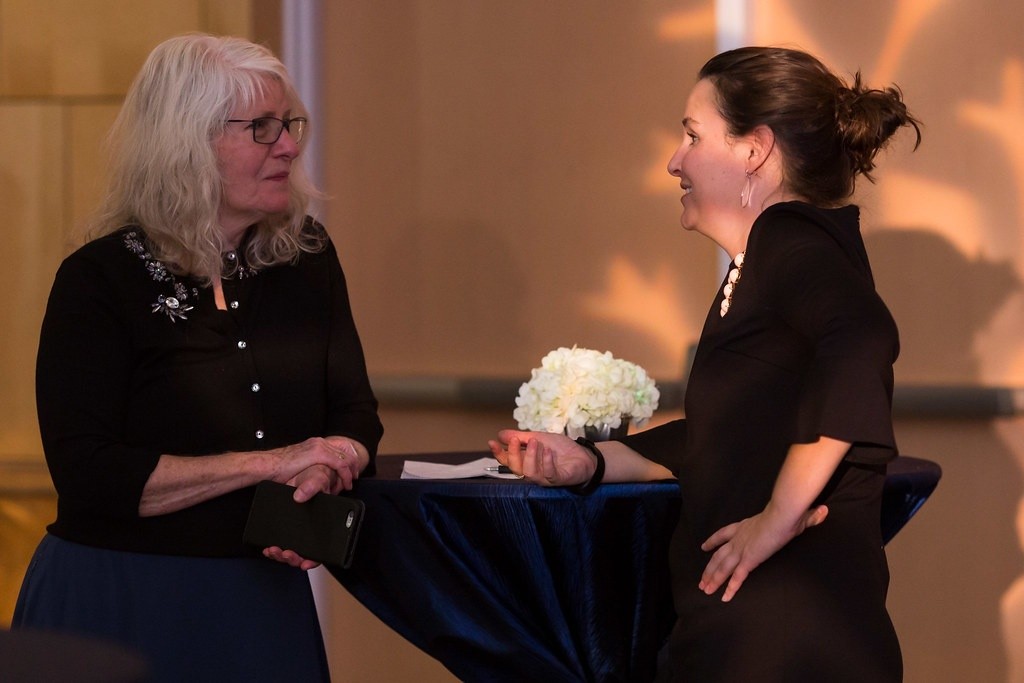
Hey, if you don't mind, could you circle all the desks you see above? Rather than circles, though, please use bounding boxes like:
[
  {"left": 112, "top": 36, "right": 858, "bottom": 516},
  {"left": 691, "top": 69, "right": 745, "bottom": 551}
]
[{"left": 323, "top": 452, "right": 944, "bottom": 683}]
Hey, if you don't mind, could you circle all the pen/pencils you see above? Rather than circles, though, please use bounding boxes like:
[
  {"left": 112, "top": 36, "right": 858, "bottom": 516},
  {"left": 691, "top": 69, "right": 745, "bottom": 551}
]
[{"left": 482, "top": 464, "right": 514, "bottom": 474}]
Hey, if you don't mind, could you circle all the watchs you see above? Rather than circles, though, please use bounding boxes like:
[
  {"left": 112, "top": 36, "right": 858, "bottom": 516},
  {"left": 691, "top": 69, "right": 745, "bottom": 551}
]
[{"left": 566, "top": 436, "right": 605, "bottom": 496}]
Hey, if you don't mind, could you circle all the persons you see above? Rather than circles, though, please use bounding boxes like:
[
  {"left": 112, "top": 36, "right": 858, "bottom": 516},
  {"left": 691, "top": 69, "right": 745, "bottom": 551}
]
[
  {"left": 486, "top": 47, "right": 922, "bottom": 683},
  {"left": 6, "top": 34, "right": 385, "bottom": 683}
]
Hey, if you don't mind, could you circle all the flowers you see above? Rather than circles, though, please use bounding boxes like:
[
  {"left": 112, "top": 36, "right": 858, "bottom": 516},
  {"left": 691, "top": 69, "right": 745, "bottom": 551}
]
[{"left": 514, "top": 346, "right": 660, "bottom": 439}]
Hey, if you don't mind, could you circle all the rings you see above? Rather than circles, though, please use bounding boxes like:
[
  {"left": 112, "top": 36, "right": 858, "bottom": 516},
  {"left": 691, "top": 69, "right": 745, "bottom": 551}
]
[
  {"left": 513, "top": 473, "right": 525, "bottom": 479},
  {"left": 338, "top": 453, "right": 344, "bottom": 459}
]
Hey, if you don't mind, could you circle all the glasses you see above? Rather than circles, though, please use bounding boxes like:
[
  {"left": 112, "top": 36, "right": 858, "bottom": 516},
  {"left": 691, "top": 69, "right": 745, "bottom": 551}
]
[{"left": 220, "top": 114, "right": 308, "bottom": 146}]
